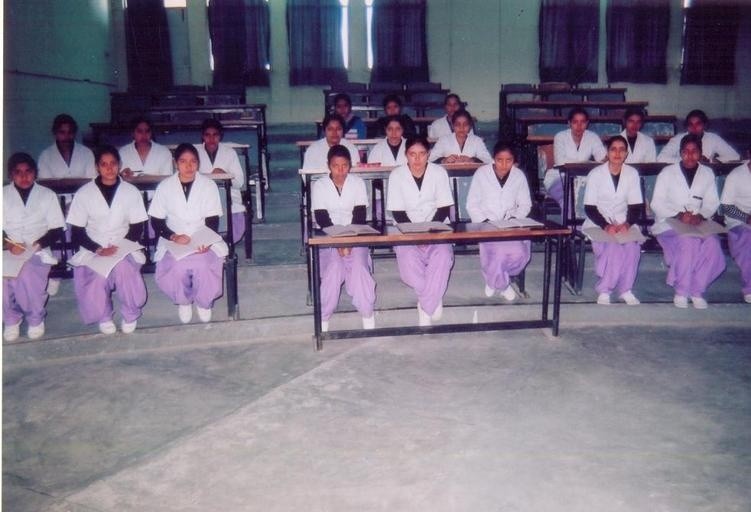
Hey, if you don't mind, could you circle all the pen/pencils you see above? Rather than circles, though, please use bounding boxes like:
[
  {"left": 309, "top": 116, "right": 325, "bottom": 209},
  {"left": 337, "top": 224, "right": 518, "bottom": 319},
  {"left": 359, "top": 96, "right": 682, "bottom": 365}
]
[
  {"left": 132, "top": 171, "right": 144, "bottom": 172},
  {"left": 683, "top": 206, "right": 688, "bottom": 212},
  {"left": 4, "top": 237, "right": 28, "bottom": 251},
  {"left": 608, "top": 216, "right": 614, "bottom": 225}
]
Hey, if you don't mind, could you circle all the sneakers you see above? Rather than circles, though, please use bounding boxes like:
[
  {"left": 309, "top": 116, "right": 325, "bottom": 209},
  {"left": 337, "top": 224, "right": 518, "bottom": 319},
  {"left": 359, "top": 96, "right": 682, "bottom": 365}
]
[
  {"left": 45, "top": 278, "right": 61, "bottom": 298},
  {"left": 673, "top": 295, "right": 689, "bottom": 309},
  {"left": 596, "top": 293, "right": 610, "bottom": 305},
  {"left": 432, "top": 299, "right": 444, "bottom": 322},
  {"left": 619, "top": 290, "right": 640, "bottom": 305},
  {"left": 120, "top": 319, "right": 137, "bottom": 334},
  {"left": 501, "top": 286, "right": 518, "bottom": 301},
  {"left": 362, "top": 313, "right": 375, "bottom": 330},
  {"left": 99, "top": 320, "right": 117, "bottom": 336},
  {"left": 3, "top": 320, "right": 24, "bottom": 343},
  {"left": 485, "top": 285, "right": 496, "bottom": 298},
  {"left": 742, "top": 294, "right": 749, "bottom": 303},
  {"left": 690, "top": 294, "right": 708, "bottom": 310},
  {"left": 197, "top": 304, "right": 212, "bottom": 322},
  {"left": 178, "top": 303, "right": 193, "bottom": 323},
  {"left": 27, "top": 314, "right": 47, "bottom": 341},
  {"left": 416, "top": 302, "right": 434, "bottom": 332}
]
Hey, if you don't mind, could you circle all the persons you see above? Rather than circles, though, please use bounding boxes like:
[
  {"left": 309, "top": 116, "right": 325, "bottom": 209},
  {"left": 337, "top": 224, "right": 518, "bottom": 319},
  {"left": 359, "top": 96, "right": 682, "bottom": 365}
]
[
  {"left": 386, "top": 135, "right": 456, "bottom": 325},
  {"left": 582, "top": 135, "right": 645, "bottom": 306},
  {"left": 303, "top": 114, "right": 361, "bottom": 170},
  {"left": 368, "top": 95, "right": 418, "bottom": 137},
  {"left": 649, "top": 134, "right": 726, "bottom": 310},
  {"left": 3, "top": 152, "right": 68, "bottom": 341},
  {"left": 147, "top": 142, "right": 230, "bottom": 324},
  {"left": 466, "top": 140, "right": 532, "bottom": 301},
  {"left": 36, "top": 114, "right": 96, "bottom": 295},
  {"left": 65, "top": 144, "right": 149, "bottom": 334},
  {"left": 118, "top": 116, "right": 173, "bottom": 262},
  {"left": 656, "top": 110, "right": 741, "bottom": 163},
  {"left": 428, "top": 95, "right": 474, "bottom": 137},
  {"left": 193, "top": 119, "right": 246, "bottom": 247},
  {"left": 429, "top": 110, "right": 491, "bottom": 164},
  {"left": 311, "top": 145, "right": 377, "bottom": 330},
  {"left": 619, "top": 107, "right": 656, "bottom": 163},
  {"left": 320, "top": 94, "right": 365, "bottom": 139},
  {"left": 366, "top": 116, "right": 408, "bottom": 165},
  {"left": 543, "top": 108, "right": 607, "bottom": 225},
  {"left": 719, "top": 159, "right": 751, "bottom": 303}
]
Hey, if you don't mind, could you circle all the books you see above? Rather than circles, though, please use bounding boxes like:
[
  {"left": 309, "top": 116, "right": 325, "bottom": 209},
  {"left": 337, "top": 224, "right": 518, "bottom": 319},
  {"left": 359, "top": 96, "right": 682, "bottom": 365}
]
[
  {"left": 397, "top": 221, "right": 454, "bottom": 234},
  {"left": 488, "top": 217, "right": 543, "bottom": 228},
  {"left": 323, "top": 224, "right": 381, "bottom": 237}
]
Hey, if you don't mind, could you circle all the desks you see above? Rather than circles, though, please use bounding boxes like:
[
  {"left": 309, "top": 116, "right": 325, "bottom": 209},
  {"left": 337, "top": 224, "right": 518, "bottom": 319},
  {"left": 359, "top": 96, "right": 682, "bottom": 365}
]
[{"left": 306, "top": 219, "right": 577, "bottom": 352}]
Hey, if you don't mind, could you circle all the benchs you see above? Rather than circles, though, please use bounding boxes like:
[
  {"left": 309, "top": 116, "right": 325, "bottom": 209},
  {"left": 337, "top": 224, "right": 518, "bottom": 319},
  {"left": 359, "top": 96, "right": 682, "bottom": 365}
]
[
  {"left": 498, "top": 80, "right": 751, "bottom": 296},
  {"left": 293, "top": 78, "right": 534, "bottom": 306},
  {"left": 3, "top": 87, "right": 271, "bottom": 322}
]
